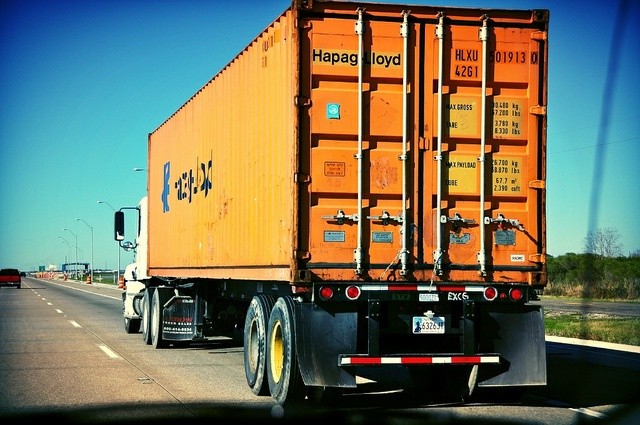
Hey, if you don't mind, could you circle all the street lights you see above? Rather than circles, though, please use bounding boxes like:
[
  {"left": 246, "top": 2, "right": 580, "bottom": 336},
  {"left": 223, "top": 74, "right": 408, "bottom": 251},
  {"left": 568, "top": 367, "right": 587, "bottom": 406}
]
[
  {"left": 64, "top": 229, "right": 78, "bottom": 279},
  {"left": 133, "top": 168, "right": 148, "bottom": 172},
  {"left": 97, "top": 201, "right": 120, "bottom": 285},
  {"left": 57, "top": 236, "right": 69, "bottom": 273},
  {"left": 72, "top": 245, "right": 83, "bottom": 281},
  {"left": 75, "top": 218, "right": 93, "bottom": 282}
]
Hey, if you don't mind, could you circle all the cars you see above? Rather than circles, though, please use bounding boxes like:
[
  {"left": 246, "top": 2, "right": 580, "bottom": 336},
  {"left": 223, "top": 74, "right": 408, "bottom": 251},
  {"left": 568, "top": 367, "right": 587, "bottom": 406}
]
[{"left": 0, "top": 269, "right": 24, "bottom": 289}]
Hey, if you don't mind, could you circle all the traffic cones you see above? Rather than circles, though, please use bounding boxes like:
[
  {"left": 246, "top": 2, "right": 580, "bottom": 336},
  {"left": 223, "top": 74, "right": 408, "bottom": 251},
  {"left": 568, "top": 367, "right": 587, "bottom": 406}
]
[
  {"left": 86, "top": 276, "right": 91, "bottom": 284},
  {"left": 118, "top": 277, "right": 124, "bottom": 288}
]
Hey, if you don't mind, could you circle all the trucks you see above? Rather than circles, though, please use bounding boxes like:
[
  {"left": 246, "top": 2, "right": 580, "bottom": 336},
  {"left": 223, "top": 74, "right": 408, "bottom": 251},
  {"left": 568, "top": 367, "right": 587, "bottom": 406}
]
[{"left": 114, "top": 1, "right": 550, "bottom": 410}]
